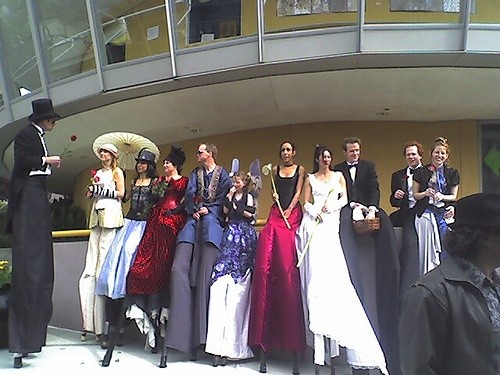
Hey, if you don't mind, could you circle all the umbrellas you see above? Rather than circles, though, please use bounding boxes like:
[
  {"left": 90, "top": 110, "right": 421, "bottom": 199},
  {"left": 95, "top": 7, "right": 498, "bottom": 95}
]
[{"left": 92, "top": 131, "right": 161, "bottom": 171}]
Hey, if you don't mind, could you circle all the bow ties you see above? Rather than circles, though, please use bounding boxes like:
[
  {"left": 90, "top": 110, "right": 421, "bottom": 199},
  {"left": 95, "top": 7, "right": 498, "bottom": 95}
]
[
  {"left": 347, "top": 164, "right": 359, "bottom": 169},
  {"left": 32, "top": 124, "right": 45, "bottom": 137},
  {"left": 409, "top": 167, "right": 421, "bottom": 174}
]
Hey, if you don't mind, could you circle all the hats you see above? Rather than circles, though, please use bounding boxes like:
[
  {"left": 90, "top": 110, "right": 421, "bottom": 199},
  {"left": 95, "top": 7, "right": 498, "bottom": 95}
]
[
  {"left": 97, "top": 143, "right": 118, "bottom": 158},
  {"left": 28, "top": 99, "right": 60, "bottom": 121},
  {"left": 135, "top": 150, "right": 156, "bottom": 168},
  {"left": 447, "top": 193, "right": 500, "bottom": 230}
]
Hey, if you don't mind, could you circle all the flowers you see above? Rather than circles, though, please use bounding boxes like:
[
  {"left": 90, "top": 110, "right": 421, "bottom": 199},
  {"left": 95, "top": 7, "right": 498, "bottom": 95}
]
[
  {"left": 318, "top": 206, "right": 328, "bottom": 218},
  {"left": 0, "top": 260, "right": 12, "bottom": 285},
  {"left": 60, "top": 135, "right": 77, "bottom": 158},
  {"left": 428, "top": 166, "right": 437, "bottom": 203},
  {"left": 147, "top": 176, "right": 169, "bottom": 200},
  {"left": 83, "top": 170, "right": 100, "bottom": 194},
  {"left": 402, "top": 175, "right": 409, "bottom": 191},
  {"left": 263, "top": 163, "right": 291, "bottom": 231}
]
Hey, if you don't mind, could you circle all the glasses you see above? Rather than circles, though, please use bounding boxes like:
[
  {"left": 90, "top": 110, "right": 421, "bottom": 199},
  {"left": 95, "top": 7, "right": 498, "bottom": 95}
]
[{"left": 195, "top": 150, "right": 209, "bottom": 156}]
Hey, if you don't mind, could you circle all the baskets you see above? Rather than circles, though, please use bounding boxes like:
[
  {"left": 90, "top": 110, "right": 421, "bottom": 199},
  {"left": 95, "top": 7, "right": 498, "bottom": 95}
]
[{"left": 352, "top": 208, "right": 380, "bottom": 234}]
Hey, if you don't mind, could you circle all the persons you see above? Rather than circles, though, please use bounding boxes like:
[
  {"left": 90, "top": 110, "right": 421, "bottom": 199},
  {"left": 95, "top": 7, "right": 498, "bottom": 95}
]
[
  {"left": 390, "top": 141, "right": 424, "bottom": 288},
  {"left": 251, "top": 141, "right": 305, "bottom": 310},
  {"left": 296, "top": 146, "right": 362, "bottom": 313},
  {"left": 167, "top": 141, "right": 232, "bottom": 310},
  {"left": 400, "top": 193, "right": 500, "bottom": 375},
  {"left": 5, "top": 99, "right": 61, "bottom": 307},
  {"left": 96, "top": 151, "right": 158, "bottom": 300},
  {"left": 126, "top": 150, "right": 189, "bottom": 295},
  {"left": 413, "top": 137, "right": 460, "bottom": 277},
  {"left": 333, "top": 137, "right": 402, "bottom": 327},
  {"left": 77, "top": 144, "right": 125, "bottom": 297},
  {"left": 209, "top": 172, "right": 257, "bottom": 328}
]
